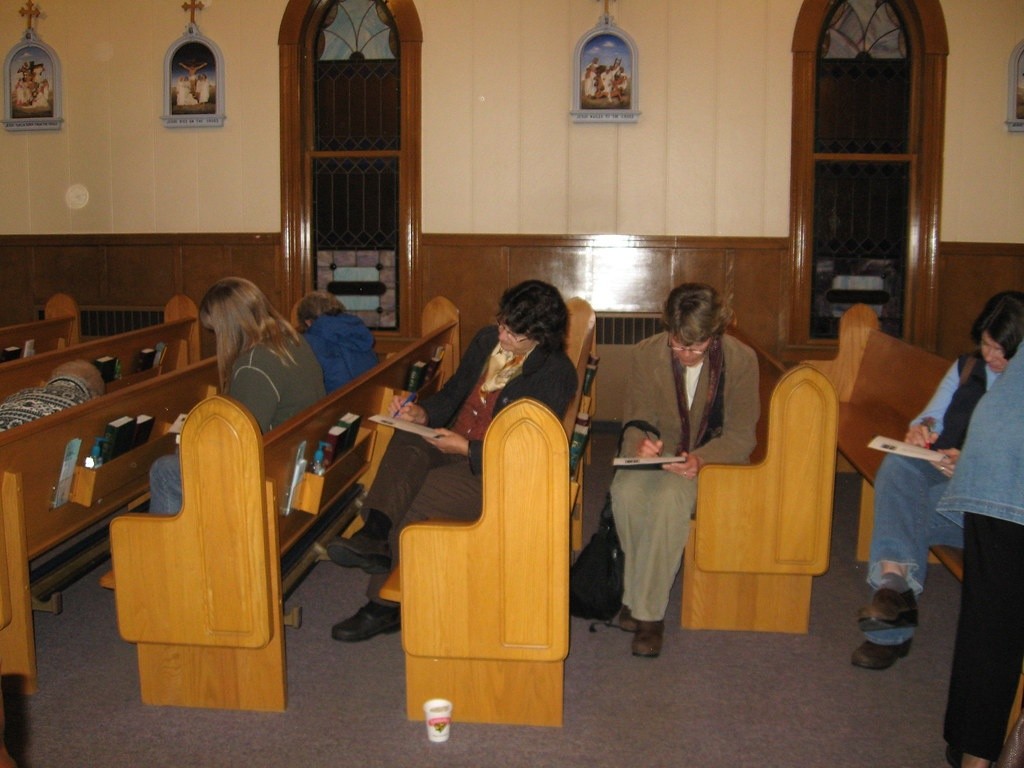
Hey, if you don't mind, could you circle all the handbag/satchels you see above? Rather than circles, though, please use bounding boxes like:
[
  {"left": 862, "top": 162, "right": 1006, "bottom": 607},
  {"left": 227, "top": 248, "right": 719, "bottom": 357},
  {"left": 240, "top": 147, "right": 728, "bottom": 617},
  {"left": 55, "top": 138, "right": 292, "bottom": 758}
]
[{"left": 569, "top": 516, "right": 627, "bottom": 621}]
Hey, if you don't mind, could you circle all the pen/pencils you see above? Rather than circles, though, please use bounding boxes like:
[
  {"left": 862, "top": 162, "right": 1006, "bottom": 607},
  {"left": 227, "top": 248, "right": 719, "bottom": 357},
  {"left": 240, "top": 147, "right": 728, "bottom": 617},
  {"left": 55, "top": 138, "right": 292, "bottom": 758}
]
[
  {"left": 391, "top": 392, "right": 416, "bottom": 420},
  {"left": 643, "top": 427, "right": 662, "bottom": 458},
  {"left": 924, "top": 418, "right": 934, "bottom": 449},
  {"left": 159, "top": 346, "right": 168, "bottom": 364}
]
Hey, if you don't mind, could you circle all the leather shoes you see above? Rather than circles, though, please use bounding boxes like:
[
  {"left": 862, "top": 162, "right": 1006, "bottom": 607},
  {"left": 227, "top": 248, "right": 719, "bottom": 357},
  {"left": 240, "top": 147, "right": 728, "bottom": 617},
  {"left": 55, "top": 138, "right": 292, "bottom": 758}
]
[
  {"left": 325, "top": 523, "right": 391, "bottom": 574},
  {"left": 331, "top": 599, "right": 402, "bottom": 643}
]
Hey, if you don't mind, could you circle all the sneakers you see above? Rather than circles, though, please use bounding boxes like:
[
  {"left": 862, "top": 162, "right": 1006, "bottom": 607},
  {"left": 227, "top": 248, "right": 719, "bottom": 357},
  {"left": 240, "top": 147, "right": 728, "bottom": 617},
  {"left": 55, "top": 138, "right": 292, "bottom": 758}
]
[
  {"left": 858, "top": 587, "right": 918, "bottom": 632},
  {"left": 630, "top": 621, "right": 666, "bottom": 655},
  {"left": 851, "top": 639, "right": 911, "bottom": 669},
  {"left": 620, "top": 605, "right": 637, "bottom": 631}
]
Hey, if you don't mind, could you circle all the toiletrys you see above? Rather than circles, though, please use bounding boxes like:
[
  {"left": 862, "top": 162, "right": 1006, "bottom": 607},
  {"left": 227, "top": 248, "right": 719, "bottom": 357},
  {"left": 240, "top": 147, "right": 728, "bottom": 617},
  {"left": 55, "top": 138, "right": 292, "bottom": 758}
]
[
  {"left": 310, "top": 439, "right": 331, "bottom": 475},
  {"left": 85, "top": 435, "right": 110, "bottom": 468}
]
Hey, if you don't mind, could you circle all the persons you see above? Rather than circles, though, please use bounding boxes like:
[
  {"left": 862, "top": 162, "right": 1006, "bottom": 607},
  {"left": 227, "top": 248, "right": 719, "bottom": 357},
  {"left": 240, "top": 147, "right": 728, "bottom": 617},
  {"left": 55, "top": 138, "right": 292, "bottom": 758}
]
[
  {"left": 850, "top": 291, "right": 1024, "bottom": 768},
  {"left": 291, "top": 291, "right": 379, "bottom": 395},
  {"left": 150, "top": 277, "right": 326, "bottom": 514},
  {"left": 327, "top": 279, "right": 579, "bottom": 642},
  {"left": 610, "top": 282, "right": 760, "bottom": 658}
]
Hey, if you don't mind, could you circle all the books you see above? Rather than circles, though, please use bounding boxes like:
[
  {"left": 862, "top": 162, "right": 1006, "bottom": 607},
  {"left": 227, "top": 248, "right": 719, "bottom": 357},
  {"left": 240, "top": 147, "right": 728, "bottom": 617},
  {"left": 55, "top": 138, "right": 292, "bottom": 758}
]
[
  {"left": 569, "top": 395, "right": 592, "bottom": 478},
  {"left": 95, "top": 355, "right": 117, "bottom": 384},
  {"left": 0, "top": 346, "right": 21, "bottom": 364},
  {"left": 139, "top": 348, "right": 157, "bottom": 372},
  {"left": 408, "top": 350, "right": 445, "bottom": 392},
  {"left": 102, "top": 413, "right": 153, "bottom": 463},
  {"left": 328, "top": 412, "right": 362, "bottom": 468}
]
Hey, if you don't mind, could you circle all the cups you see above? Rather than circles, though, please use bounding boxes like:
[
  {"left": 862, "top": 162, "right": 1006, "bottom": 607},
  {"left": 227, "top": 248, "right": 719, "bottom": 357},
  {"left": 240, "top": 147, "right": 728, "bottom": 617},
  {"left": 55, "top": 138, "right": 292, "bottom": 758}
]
[{"left": 423, "top": 698, "right": 453, "bottom": 743}]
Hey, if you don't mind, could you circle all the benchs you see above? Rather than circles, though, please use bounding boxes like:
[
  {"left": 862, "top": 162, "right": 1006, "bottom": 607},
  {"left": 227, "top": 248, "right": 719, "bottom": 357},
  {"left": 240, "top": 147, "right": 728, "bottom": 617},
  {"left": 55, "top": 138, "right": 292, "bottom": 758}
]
[
  {"left": 680, "top": 321, "right": 839, "bottom": 635},
  {"left": 383, "top": 295, "right": 594, "bottom": 727},
  {"left": 107, "top": 295, "right": 458, "bottom": 715},
  {"left": 0, "top": 294, "right": 82, "bottom": 349},
  {"left": 804, "top": 305, "right": 1024, "bottom": 744},
  {"left": 0, "top": 298, "right": 199, "bottom": 405},
  {"left": 0, "top": 298, "right": 306, "bottom": 694}
]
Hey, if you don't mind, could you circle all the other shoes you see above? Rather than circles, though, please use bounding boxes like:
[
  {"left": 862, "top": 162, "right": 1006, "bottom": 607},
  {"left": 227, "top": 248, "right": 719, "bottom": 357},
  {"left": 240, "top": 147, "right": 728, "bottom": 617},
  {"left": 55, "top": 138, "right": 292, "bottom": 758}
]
[{"left": 98, "top": 569, "right": 114, "bottom": 589}]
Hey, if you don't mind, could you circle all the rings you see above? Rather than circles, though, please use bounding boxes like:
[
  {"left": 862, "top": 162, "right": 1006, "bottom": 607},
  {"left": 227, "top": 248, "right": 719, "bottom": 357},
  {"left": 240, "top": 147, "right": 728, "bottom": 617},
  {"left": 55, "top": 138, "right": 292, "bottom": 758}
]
[
  {"left": 683, "top": 471, "right": 686, "bottom": 476},
  {"left": 938, "top": 465, "right": 944, "bottom": 472}
]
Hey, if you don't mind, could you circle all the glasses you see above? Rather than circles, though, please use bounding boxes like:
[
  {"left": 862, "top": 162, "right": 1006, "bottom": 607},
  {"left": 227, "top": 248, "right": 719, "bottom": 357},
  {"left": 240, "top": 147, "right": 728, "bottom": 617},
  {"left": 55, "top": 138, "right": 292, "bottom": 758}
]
[
  {"left": 667, "top": 330, "right": 709, "bottom": 354},
  {"left": 495, "top": 312, "right": 528, "bottom": 344}
]
